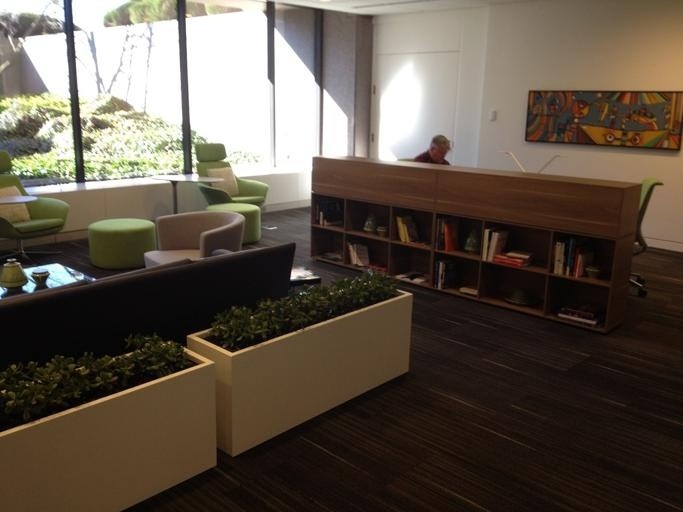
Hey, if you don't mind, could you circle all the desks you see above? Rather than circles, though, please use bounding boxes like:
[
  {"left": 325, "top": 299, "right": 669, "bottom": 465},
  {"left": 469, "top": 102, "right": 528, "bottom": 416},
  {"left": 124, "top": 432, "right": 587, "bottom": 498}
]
[
  {"left": 0, "top": 195, "right": 38, "bottom": 207},
  {"left": 149, "top": 173, "right": 224, "bottom": 214},
  {"left": 0, "top": 260, "right": 96, "bottom": 302}
]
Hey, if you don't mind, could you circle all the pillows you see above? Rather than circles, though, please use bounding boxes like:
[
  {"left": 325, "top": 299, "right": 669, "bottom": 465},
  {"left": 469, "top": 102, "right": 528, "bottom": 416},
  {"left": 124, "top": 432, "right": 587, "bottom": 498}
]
[
  {"left": 0, "top": 185, "right": 31, "bottom": 224},
  {"left": 204, "top": 166, "right": 240, "bottom": 197}
]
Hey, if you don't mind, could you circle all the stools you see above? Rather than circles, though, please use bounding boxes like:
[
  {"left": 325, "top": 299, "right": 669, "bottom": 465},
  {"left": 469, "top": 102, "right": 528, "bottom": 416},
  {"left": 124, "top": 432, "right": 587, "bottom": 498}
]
[
  {"left": 86, "top": 217, "right": 157, "bottom": 270},
  {"left": 205, "top": 201, "right": 261, "bottom": 245}
]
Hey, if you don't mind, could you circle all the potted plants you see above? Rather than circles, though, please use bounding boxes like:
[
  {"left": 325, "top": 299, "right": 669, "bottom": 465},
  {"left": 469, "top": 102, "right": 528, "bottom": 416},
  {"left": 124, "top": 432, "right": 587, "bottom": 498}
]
[
  {"left": 0, "top": 329, "right": 217, "bottom": 512},
  {"left": 184, "top": 266, "right": 419, "bottom": 462}
]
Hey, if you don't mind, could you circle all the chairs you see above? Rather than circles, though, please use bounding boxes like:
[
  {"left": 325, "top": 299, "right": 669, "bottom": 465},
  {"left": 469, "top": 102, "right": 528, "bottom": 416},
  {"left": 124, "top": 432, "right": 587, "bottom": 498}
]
[
  {"left": 628, "top": 174, "right": 664, "bottom": 299},
  {"left": 0, "top": 150, "right": 70, "bottom": 266},
  {"left": 142, "top": 208, "right": 245, "bottom": 268},
  {"left": 195, "top": 141, "right": 269, "bottom": 207}
]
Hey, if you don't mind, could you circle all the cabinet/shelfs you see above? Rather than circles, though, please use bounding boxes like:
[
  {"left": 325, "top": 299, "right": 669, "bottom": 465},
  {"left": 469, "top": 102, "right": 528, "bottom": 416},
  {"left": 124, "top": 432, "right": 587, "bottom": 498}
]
[{"left": 308, "top": 154, "right": 642, "bottom": 333}]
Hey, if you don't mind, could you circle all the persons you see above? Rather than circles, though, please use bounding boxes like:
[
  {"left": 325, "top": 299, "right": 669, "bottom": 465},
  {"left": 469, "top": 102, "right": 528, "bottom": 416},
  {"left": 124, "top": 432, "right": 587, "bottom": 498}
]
[
  {"left": 312, "top": 194, "right": 608, "bottom": 329},
  {"left": 414, "top": 135, "right": 451, "bottom": 166}
]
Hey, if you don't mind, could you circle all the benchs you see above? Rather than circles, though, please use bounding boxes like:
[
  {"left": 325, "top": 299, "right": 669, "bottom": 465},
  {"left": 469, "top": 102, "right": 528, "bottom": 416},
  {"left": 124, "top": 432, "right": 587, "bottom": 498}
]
[{"left": 0, "top": 241, "right": 297, "bottom": 387}]
